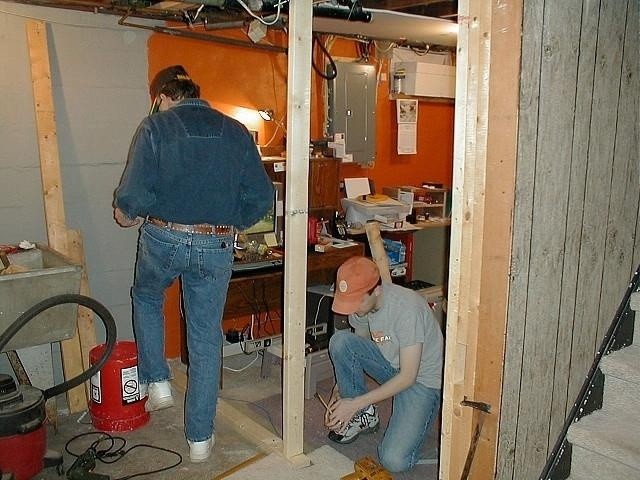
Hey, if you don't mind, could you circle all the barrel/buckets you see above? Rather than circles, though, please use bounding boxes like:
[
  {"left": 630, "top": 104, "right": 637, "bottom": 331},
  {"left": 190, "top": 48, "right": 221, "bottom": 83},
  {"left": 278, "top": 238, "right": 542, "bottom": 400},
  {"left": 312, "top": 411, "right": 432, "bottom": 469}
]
[{"left": 91, "top": 340, "right": 149, "bottom": 430}]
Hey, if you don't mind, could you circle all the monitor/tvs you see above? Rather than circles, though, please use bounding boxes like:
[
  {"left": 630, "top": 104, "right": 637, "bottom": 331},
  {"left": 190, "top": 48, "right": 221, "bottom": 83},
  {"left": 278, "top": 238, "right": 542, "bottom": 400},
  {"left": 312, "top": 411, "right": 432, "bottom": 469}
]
[{"left": 233, "top": 181, "right": 285, "bottom": 247}]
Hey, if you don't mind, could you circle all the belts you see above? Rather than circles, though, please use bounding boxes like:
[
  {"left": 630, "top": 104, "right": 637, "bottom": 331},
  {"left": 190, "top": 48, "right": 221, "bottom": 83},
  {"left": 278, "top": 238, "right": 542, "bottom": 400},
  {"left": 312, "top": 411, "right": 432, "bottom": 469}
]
[{"left": 151, "top": 218, "right": 232, "bottom": 236}]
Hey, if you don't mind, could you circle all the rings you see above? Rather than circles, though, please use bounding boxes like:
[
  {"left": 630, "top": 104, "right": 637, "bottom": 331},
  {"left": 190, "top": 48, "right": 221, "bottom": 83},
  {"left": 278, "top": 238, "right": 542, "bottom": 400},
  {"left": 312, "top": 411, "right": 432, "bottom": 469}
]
[{"left": 338, "top": 421, "right": 343, "bottom": 426}]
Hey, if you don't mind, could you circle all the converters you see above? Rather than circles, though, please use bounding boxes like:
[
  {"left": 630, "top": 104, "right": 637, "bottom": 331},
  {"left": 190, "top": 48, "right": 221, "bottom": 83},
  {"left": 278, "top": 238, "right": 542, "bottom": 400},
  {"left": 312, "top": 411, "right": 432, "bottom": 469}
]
[
  {"left": 225, "top": 328, "right": 239, "bottom": 344},
  {"left": 66, "top": 452, "right": 96, "bottom": 477}
]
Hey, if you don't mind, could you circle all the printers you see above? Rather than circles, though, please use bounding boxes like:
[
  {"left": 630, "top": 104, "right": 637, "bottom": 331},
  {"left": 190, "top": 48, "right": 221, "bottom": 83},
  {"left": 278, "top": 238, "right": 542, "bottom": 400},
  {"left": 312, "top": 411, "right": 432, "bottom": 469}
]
[{"left": 340, "top": 177, "right": 411, "bottom": 227}]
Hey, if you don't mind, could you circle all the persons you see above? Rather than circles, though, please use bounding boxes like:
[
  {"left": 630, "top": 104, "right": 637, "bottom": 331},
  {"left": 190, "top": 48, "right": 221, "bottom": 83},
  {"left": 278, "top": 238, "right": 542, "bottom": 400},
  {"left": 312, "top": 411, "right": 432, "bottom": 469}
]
[
  {"left": 323, "top": 255, "right": 446, "bottom": 474},
  {"left": 111, "top": 63, "right": 276, "bottom": 462}
]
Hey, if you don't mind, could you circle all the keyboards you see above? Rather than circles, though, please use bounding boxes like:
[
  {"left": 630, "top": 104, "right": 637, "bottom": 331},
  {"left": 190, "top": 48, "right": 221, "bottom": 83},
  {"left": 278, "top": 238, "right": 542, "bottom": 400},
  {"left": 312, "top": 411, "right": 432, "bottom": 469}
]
[{"left": 231, "top": 259, "right": 283, "bottom": 279}]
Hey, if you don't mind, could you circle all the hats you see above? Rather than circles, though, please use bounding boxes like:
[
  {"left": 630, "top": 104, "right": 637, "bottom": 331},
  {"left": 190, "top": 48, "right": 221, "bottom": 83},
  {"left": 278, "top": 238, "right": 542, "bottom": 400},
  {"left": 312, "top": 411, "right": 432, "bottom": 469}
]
[
  {"left": 331, "top": 255, "right": 380, "bottom": 316},
  {"left": 149, "top": 64, "right": 192, "bottom": 115}
]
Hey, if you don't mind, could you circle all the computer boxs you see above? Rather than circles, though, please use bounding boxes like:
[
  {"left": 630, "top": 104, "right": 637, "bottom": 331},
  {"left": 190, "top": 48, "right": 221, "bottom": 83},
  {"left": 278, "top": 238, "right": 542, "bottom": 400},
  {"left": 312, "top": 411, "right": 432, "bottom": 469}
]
[{"left": 305, "top": 281, "right": 355, "bottom": 349}]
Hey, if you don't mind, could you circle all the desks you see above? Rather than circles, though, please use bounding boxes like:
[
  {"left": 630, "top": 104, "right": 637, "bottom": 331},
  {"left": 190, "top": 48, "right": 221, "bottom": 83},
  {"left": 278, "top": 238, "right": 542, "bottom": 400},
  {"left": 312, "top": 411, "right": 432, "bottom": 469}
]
[
  {"left": 336, "top": 187, "right": 451, "bottom": 281},
  {"left": 179, "top": 147, "right": 364, "bottom": 389}
]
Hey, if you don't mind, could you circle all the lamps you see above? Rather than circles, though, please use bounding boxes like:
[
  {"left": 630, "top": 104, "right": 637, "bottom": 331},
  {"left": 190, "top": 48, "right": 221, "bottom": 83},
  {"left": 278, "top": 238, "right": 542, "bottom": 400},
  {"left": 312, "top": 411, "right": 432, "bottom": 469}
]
[{"left": 259, "top": 106, "right": 287, "bottom": 140}]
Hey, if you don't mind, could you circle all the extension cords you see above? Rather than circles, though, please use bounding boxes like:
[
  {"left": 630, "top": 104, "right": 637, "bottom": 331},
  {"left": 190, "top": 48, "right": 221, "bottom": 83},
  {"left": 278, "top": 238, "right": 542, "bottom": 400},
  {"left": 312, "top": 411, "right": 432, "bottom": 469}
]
[{"left": 223, "top": 332, "right": 248, "bottom": 345}]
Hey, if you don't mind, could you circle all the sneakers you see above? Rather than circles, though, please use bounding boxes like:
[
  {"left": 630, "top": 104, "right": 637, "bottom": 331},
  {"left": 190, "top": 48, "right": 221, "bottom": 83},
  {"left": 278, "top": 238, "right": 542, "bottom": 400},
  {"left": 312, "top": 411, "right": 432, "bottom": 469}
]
[
  {"left": 328, "top": 405, "right": 380, "bottom": 444},
  {"left": 144, "top": 381, "right": 175, "bottom": 412},
  {"left": 187, "top": 432, "right": 216, "bottom": 463}
]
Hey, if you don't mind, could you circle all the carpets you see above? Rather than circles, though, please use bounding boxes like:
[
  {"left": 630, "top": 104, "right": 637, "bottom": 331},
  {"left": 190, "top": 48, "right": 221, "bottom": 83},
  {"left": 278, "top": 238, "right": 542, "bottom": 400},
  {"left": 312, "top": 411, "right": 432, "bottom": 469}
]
[{"left": 247, "top": 371, "right": 439, "bottom": 480}]
